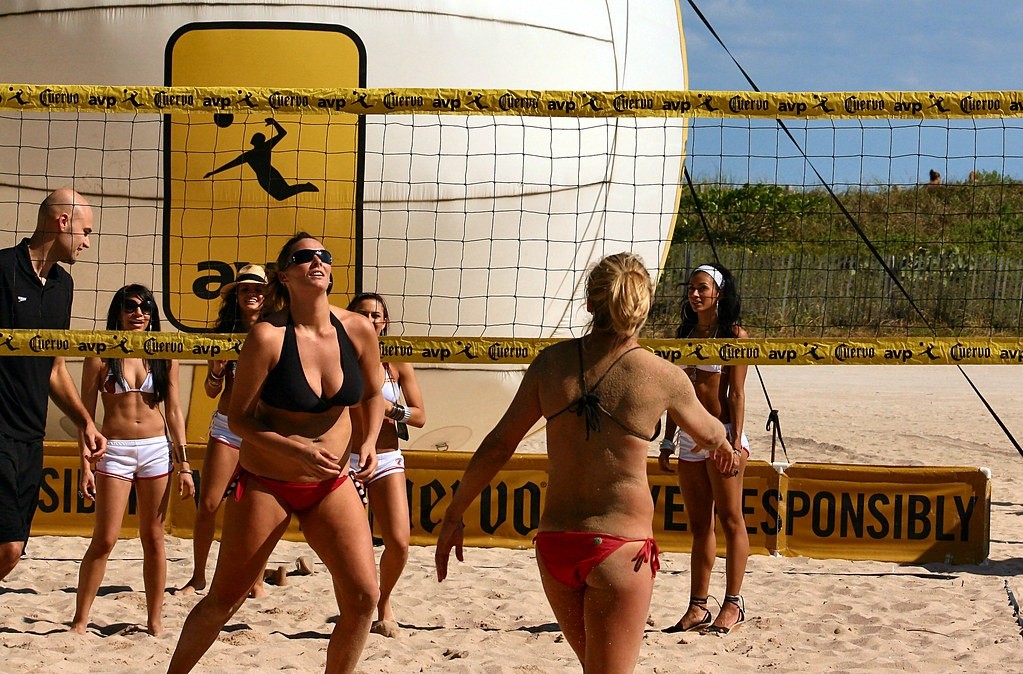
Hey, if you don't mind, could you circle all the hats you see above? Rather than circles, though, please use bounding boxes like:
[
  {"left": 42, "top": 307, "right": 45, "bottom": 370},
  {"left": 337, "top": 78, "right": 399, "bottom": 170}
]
[{"left": 220, "top": 264, "right": 270, "bottom": 300}]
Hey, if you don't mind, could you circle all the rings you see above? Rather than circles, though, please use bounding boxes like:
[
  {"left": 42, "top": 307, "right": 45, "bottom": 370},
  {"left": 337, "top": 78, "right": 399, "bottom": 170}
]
[
  {"left": 734, "top": 469, "right": 738, "bottom": 473},
  {"left": 78, "top": 491, "right": 84, "bottom": 498}
]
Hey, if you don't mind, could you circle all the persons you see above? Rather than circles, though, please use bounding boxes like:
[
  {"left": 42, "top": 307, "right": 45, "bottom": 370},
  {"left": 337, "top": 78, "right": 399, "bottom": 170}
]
[
  {"left": 69, "top": 284, "right": 196, "bottom": 637},
  {"left": 929, "top": 169, "right": 941, "bottom": 186},
  {"left": 657, "top": 262, "right": 750, "bottom": 637},
  {"left": 0, "top": 188, "right": 108, "bottom": 580},
  {"left": 167, "top": 232, "right": 386, "bottom": 674},
  {"left": 344, "top": 293, "right": 426, "bottom": 623},
  {"left": 435, "top": 251, "right": 734, "bottom": 674},
  {"left": 174, "top": 264, "right": 269, "bottom": 598}
]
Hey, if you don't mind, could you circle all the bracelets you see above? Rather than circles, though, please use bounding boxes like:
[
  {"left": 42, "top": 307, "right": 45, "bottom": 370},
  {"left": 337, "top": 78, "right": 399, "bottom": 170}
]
[
  {"left": 208, "top": 369, "right": 225, "bottom": 389},
  {"left": 734, "top": 448, "right": 742, "bottom": 457},
  {"left": 659, "top": 440, "right": 675, "bottom": 454},
  {"left": 180, "top": 469, "right": 193, "bottom": 474},
  {"left": 385, "top": 403, "right": 411, "bottom": 423}
]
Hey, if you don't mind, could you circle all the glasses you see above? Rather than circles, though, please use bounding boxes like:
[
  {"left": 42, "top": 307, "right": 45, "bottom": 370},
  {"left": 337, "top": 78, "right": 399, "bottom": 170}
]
[
  {"left": 119, "top": 299, "right": 154, "bottom": 315},
  {"left": 282, "top": 249, "right": 331, "bottom": 270}
]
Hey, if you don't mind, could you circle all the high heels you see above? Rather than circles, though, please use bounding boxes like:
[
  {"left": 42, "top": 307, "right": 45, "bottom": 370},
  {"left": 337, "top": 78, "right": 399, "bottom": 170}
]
[
  {"left": 699, "top": 595, "right": 745, "bottom": 636},
  {"left": 661, "top": 595, "right": 722, "bottom": 633},
  {"left": 263, "top": 566, "right": 287, "bottom": 586},
  {"left": 294, "top": 555, "right": 327, "bottom": 575}
]
